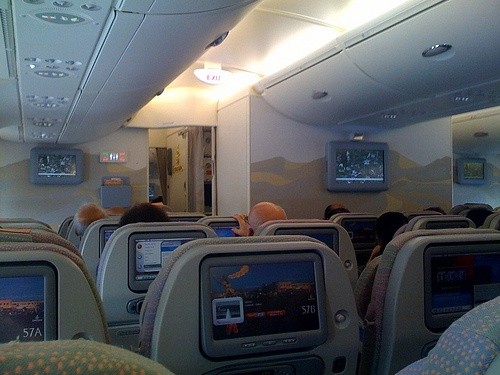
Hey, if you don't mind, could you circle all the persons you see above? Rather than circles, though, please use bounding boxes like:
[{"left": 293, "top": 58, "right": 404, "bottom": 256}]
[
  {"left": 231, "top": 201, "right": 288, "bottom": 236},
  {"left": 73, "top": 203, "right": 108, "bottom": 250},
  {"left": 117, "top": 203, "right": 172, "bottom": 228},
  {"left": 366, "top": 211, "right": 410, "bottom": 265},
  {"left": 324, "top": 203, "right": 350, "bottom": 220}
]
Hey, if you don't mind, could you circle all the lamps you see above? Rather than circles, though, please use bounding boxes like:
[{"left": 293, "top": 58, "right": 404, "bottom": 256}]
[{"left": 194, "top": 62, "right": 233, "bottom": 86}]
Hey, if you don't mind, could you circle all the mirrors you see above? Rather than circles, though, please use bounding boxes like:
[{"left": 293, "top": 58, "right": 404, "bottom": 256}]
[{"left": 452, "top": 105, "right": 500, "bottom": 211}]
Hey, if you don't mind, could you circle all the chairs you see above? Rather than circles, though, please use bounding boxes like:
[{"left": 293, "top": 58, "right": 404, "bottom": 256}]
[{"left": 1, "top": 203, "right": 500, "bottom": 375}]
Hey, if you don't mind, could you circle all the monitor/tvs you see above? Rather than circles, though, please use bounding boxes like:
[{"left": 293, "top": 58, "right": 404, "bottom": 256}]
[
  {"left": 201, "top": 253, "right": 328, "bottom": 357},
  {"left": 0, "top": 266, "right": 57, "bottom": 344},
  {"left": 68, "top": 214, "right": 500, "bottom": 292},
  {"left": 324, "top": 141, "right": 389, "bottom": 193},
  {"left": 456, "top": 158, "right": 488, "bottom": 185},
  {"left": 30, "top": 147, "right": 83, "bottom": 185},
  {"left": 424, "top": 245, "right": 500, "bottom": 332}
]
